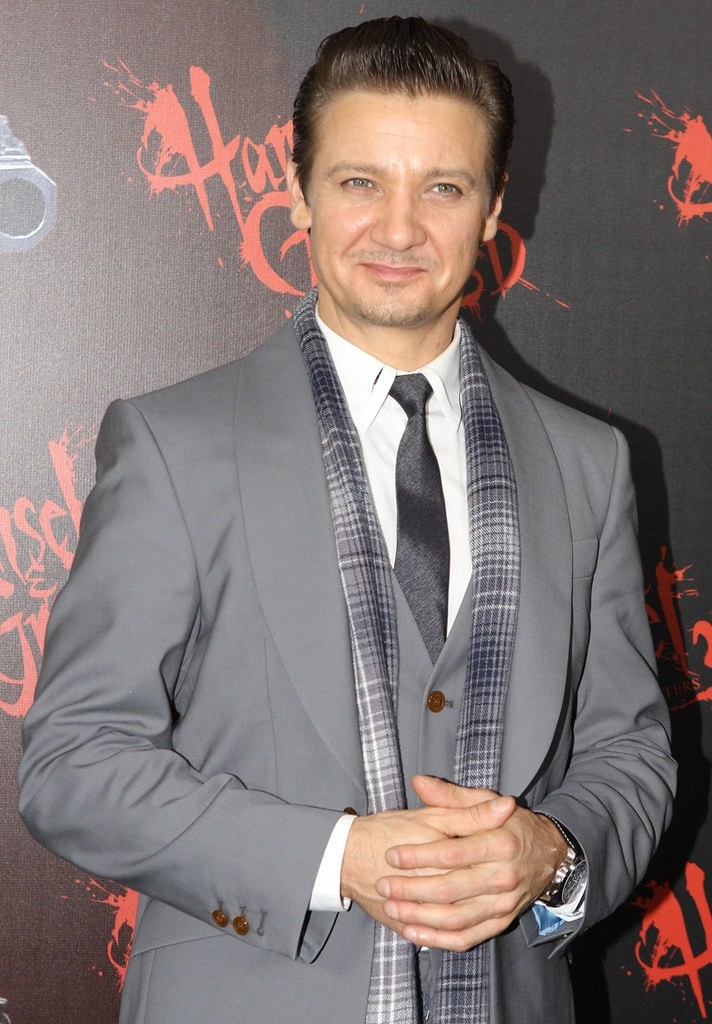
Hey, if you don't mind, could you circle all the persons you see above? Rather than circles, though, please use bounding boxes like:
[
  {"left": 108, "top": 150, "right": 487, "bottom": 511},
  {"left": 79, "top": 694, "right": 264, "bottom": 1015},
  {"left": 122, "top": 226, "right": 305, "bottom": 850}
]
[{"left": 14, "top": 14, "right": 681, "bottom": 1024}]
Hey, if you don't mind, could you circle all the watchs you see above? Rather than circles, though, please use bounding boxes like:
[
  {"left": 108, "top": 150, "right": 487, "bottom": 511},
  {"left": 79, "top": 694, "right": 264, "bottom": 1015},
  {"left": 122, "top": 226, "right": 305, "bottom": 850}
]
[{"left": 533, "top": 812, "right": 591, "bottom": 914}]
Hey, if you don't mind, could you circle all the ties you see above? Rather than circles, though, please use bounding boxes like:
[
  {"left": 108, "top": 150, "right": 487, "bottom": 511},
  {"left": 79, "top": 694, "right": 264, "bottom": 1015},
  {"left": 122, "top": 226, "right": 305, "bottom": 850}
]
[{"left": 388, "top": 373, "right": 450, "bottom": 667}]
